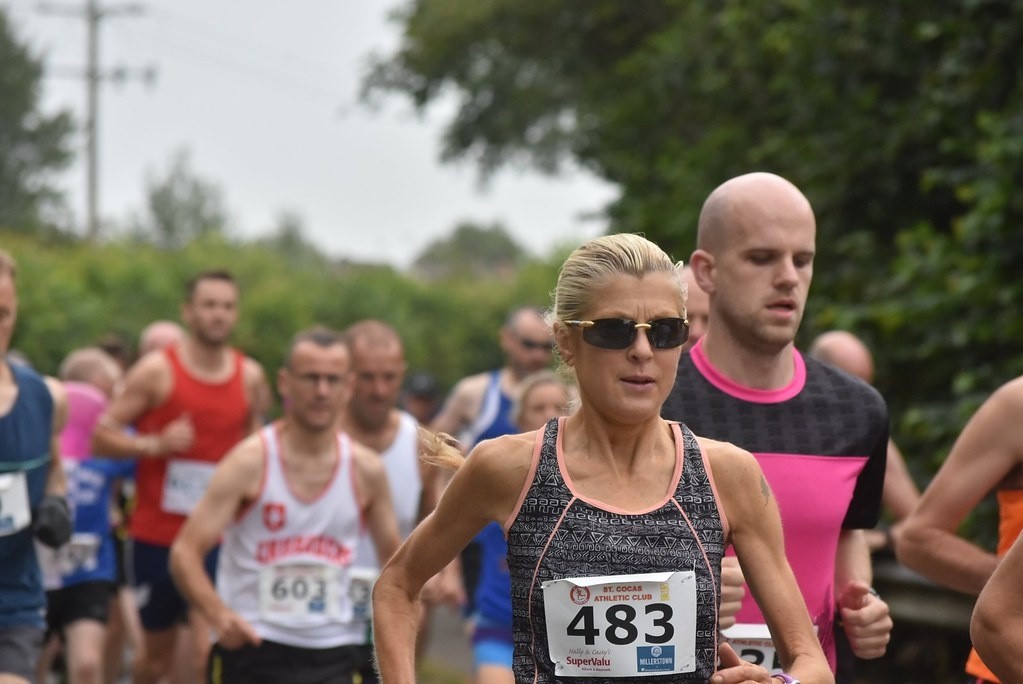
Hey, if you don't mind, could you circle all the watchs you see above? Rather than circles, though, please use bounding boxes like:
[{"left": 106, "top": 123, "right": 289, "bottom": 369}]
[{"left": 771, "top": 673, "right": 803, "bottom": 684}]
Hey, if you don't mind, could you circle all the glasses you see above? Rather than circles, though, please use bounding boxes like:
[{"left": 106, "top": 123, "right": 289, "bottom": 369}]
[
  {"left": 563, "top": 306, "right": 689, "bottom": 349},
  {"left": 522, "top": 339, "right": 554, "bottom": 351}
]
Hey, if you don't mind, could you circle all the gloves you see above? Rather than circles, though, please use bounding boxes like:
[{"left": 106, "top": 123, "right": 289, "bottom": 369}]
[{"left": 29, "top": 495, "right": 72, "bottom": 548}]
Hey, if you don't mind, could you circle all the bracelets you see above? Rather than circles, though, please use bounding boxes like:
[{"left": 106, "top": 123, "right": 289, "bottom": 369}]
[
  {"left": 867, "top": 585, "right": 883, "bottom": 601},
  {"left": 881, "top": 525, "right": 893, "bottom": 548}
]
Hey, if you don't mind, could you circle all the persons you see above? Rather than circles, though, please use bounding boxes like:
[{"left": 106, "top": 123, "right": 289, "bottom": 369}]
[
  {"left": 895, "top": 377, "right": 1023, "bottom": 684},
  {"left": 0, "top": 248, "right": 142, "bottom": 684},
  {"left": 653, "top": 171, "right": 921, "bottom": 684},
  {"left": 55, "top": 268, "right": 272, "bottom": 684},
  {"left": 423, "top": 303, "right": 557, "bottom": 637},
  {"left": 398, "top": 373, "right": 445, "bottom": 431},
  {"left": 370, "top": 238, "right": 836, "bottom": 684},
  {"left": 169, "top": 325, "right": 425, "bottom": 684},
  {"left": 334, "top": 316, "right": 468, "bottom": 610},
  {"left": 453, "top": 376, "right": 575, "bottom": 684}
]
